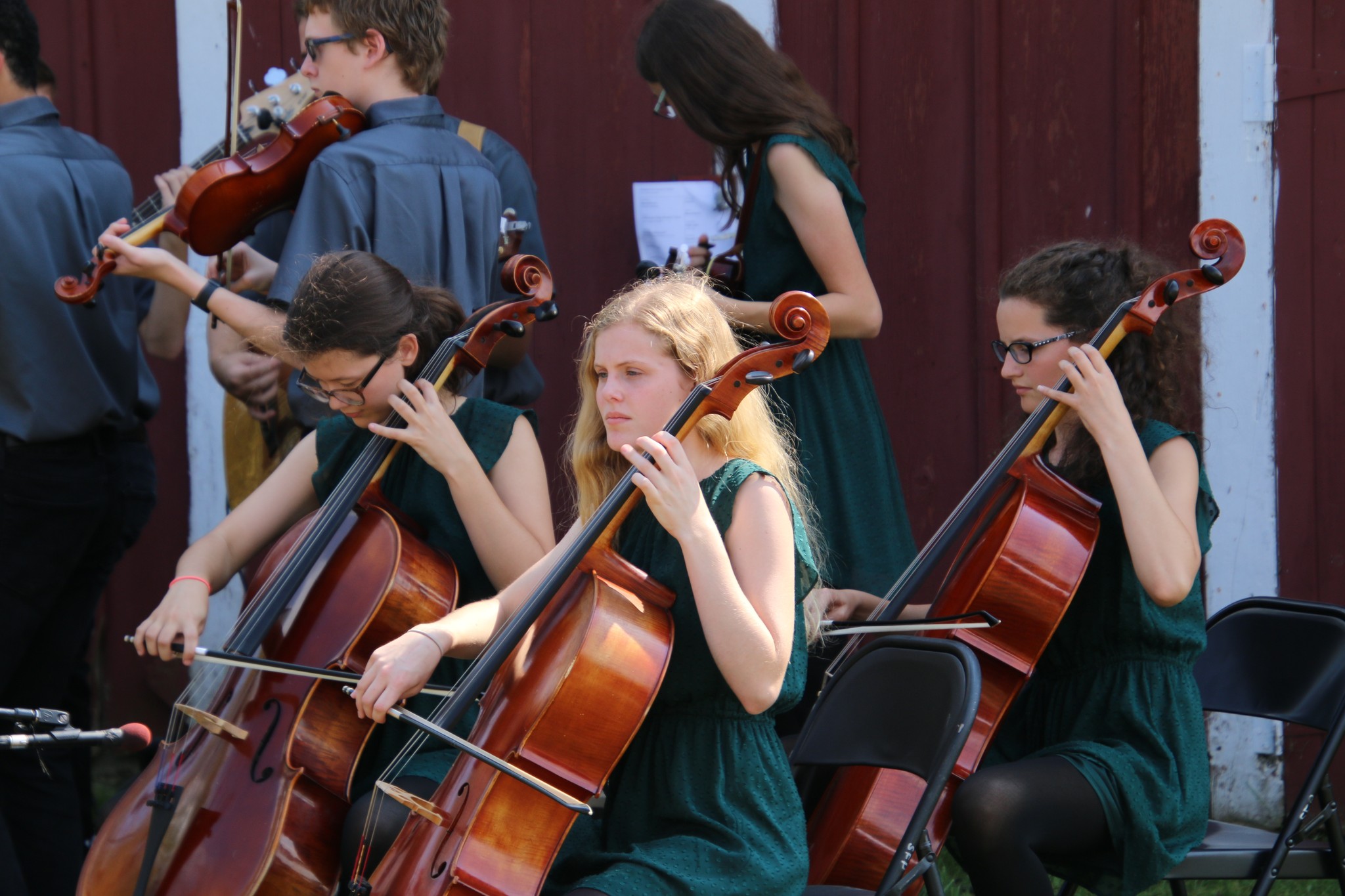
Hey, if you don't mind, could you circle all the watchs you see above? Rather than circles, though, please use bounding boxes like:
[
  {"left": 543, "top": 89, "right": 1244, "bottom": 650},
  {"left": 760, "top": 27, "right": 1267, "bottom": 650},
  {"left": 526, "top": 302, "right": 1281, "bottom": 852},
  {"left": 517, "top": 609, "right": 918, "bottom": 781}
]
[{"left": 190, "top": 277, "right": 223, "bottom": 314}]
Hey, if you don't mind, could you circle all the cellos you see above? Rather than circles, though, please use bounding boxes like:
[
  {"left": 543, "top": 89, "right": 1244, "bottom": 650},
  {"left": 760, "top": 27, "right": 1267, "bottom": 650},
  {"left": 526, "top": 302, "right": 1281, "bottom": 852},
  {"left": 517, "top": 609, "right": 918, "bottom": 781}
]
[
  {"left": 788, "top": 216, "right": 1247, "bottom": 896},
  {"left": 346, "top": 290, "right": 832, "bottom": 896},
  {"left": 73, "top": 249, "right": 558, "bottom": 896}
]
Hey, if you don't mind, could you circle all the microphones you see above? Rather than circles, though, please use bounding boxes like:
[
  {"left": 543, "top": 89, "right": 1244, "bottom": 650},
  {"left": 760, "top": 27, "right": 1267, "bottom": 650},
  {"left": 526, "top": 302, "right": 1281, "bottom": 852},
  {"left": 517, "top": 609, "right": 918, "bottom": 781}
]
[{"left": 0, "top": 723, "right": 151, "bottom": 752}]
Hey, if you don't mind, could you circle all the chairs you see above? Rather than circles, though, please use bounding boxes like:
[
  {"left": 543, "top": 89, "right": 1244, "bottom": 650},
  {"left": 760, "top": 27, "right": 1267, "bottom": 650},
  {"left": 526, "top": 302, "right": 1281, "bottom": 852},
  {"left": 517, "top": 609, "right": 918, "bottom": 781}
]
[
  {"left": 1043, "top": 595, "right": 1345, "bottom": 896},
  {"left": 784, "top": 635, "right": 981, "bottom": 896}
]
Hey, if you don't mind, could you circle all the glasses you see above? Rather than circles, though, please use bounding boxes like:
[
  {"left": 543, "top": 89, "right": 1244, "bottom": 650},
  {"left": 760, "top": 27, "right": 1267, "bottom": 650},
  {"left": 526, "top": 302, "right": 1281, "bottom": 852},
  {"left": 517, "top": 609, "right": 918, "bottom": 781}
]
[
  {"left": 296, "top": 348, "right": 397, "bottom": 407},
  {"left": 304, "top": 32, "right": 393, "bottom": 62},
  {"left": 654, "top": 90, "right": 677, "bottom": 120},
  {"left": 991, "top": 324, "right": 1100, "bottom": 364}
]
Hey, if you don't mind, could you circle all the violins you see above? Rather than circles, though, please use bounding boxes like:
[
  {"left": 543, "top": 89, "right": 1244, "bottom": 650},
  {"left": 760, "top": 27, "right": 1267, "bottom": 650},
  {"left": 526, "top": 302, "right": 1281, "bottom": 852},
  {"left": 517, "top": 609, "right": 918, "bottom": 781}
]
[{"left": 51, "top": 89, "right": 364, "bottom": 306}]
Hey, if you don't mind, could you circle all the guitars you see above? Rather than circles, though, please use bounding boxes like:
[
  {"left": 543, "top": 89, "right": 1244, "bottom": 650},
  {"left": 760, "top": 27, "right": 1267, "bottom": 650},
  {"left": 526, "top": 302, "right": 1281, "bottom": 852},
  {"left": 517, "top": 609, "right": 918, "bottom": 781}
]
[{"left": 130, "top": 64, "right": 321, "bottom": 232}]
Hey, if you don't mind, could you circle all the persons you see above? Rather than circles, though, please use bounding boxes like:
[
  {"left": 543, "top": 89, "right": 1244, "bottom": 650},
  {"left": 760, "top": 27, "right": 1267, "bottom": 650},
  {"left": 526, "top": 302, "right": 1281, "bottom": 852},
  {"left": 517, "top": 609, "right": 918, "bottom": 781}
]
[
  {"left": 801, "top": 238, "right": 1221, "bottom": 896},
  {"left": 92, "top": 0, "right": 550, "bottom": 464},
  {"left": 133, "top": 252, "right": 824, "bottom": 896},
  {"left": 633, "top": 0, "right": 918, "bottom": 632},
  {"left": 0, "top": 0, "right": 197, "bottom": 895}
]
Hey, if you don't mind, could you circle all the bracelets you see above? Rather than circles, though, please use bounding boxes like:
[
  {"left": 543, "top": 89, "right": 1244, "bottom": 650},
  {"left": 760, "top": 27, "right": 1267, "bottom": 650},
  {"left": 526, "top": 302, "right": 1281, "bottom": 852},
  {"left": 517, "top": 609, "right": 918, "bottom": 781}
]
[
  {"left": 407, "top": 629, "right": 444, "bottom": 657},
  {"left": 169, "top": 576, "right": 211, "bottom": 593}
]
